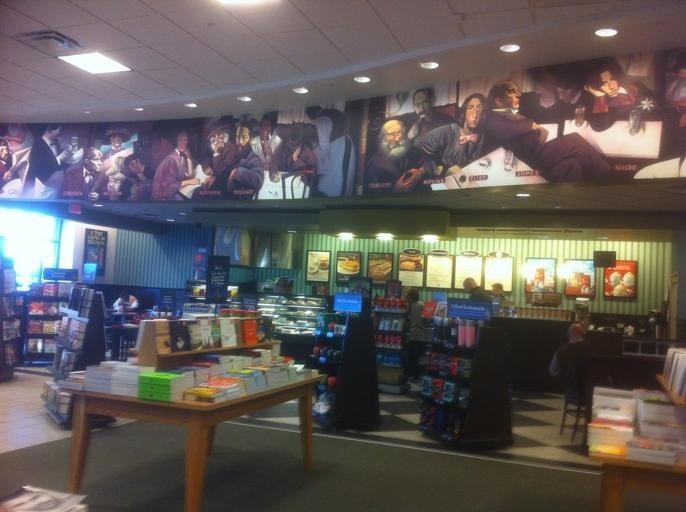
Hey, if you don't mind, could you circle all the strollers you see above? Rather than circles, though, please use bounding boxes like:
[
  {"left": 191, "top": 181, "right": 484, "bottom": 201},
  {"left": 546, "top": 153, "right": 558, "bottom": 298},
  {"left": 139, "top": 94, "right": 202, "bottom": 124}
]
[
  {"left": 85, "top": 171, "right": 94, "bottom": 177},
  {"left": 459, "top": 133, "right": 480, "bottom": 145},
  {"left": 50, "top": 142, "right": 58, "bottom": 146}
]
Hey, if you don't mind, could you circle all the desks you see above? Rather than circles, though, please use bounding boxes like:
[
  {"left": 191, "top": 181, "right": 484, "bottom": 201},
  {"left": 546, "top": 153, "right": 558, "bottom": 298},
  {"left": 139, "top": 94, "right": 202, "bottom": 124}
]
[
  {"left": 616, "top": 338, "right": 685, "bottom": 361},
  {"left": 61, "top": 320, "right": 325, "bottom": 512},
  {"left": 589, "top": 374, "right": 686, "bottom": 512}
]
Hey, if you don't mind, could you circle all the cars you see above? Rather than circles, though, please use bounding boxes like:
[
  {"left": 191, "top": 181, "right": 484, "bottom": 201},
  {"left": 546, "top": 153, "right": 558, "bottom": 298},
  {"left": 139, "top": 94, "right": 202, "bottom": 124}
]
[
  {"left": 152, "top": 311, "right": 172, "bottom": 319},
  {"left": 518, "top": 306, "right": 571, "bottom": 321}
]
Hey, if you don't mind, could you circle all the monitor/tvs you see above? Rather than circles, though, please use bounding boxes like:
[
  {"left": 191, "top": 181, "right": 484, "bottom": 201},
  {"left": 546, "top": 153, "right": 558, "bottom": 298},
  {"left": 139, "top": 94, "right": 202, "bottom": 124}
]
[
  {"left": 0, "top": 484, "right": 88, "bottom": 512},
  {"left": 662, "top": 346, "right": 686, "bottom": 397},
  {"left": 584, "top": 388, "right": 686, "bottom": 473}
]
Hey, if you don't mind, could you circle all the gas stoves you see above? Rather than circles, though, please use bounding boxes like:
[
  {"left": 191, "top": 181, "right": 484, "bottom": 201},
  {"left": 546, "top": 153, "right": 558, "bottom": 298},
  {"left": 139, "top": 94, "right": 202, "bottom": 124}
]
[{"left": 339, "top": 261, "right": 359, "bottom": 272}]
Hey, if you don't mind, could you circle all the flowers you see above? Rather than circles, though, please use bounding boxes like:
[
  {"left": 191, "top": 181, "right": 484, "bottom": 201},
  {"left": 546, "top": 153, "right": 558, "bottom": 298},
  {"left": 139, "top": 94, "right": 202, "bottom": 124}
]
[{"left": 648, "top": 308, "right": 665, "bottom": 324}]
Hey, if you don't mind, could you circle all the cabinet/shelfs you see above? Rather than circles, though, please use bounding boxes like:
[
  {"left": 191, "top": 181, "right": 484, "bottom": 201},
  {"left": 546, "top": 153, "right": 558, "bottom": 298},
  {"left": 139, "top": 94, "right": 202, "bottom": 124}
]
[
  {"left": 309, "top": 311, "right": 382, "bottom": 429},
  {"left": 117, "top": 337, "right": 135, "bottom": 361},
  {"left": 432, "top": 316, "right": 485, "bottom": 349},
  {"left": 311, "top": 344, "right": 341, "bottom": 364},
  {"left": 38, "top": 287, "right": 118, "bottom": 426},
  {"left": 183, "top": 303, "right": 212, "bottom": 313},
  {"left": 419, "top": 302, "right": 513, "bottom": 448},
  {"left": 0, "top": 266, "right": 78, "bottom": 382},
  {"left": 184, "top": 293, "right": 412, "bottom": 395}
]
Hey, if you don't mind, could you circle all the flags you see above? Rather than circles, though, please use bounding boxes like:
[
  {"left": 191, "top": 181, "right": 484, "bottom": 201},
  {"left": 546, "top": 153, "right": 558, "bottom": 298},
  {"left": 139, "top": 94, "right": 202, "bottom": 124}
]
[{"left": 656, "top": 325, "right": 666, "bottom": 339}]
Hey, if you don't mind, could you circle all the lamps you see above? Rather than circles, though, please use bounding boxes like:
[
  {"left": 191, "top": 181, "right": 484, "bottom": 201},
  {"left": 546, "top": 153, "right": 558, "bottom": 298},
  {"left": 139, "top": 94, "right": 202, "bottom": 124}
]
[{"left": 558, "top": 338, "right": 607, "bottom": 443}]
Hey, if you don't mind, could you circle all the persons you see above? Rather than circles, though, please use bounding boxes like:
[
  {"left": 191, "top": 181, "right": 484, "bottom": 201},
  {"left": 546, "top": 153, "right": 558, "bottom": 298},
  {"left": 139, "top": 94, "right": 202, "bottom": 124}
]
[
  {"left": 405, "top": 289, "right": 424, "bottom": 347},
  {"left": 491, "top": 282, "right": 506, "bottom": 302},
  {"left": 460, "top": 277, "right": 488, "bottom": 315},
  {"left": 1, "top": 487, "right": 84, "bottom": 511}
]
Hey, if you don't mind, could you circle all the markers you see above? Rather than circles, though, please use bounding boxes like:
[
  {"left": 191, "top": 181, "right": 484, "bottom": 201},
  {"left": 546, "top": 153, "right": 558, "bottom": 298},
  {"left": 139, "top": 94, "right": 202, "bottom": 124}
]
[{"left": 610, "top": 271, "right": 634, "bottom": 296}]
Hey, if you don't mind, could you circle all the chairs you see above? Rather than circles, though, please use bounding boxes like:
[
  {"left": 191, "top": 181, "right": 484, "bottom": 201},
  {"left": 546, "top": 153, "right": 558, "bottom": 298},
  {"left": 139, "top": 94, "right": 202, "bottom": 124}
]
[{"left": 558, "top": 338, "right": 607, "bottom": 443}]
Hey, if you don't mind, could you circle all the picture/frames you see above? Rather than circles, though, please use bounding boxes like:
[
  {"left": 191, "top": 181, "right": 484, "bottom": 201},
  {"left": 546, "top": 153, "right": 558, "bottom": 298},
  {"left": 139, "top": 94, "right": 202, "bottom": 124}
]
[
  {"left": 589, "top": 374, "right": 686, "bottom": 512},
  {"left": 61, "top": 320, "right": 325, "bottom": 512},
  {"left": 307, "top": 250, "right": 638, "bottom": 302},
  {"left": 616, "top": 338, "right": 685, "bottom": 361}
]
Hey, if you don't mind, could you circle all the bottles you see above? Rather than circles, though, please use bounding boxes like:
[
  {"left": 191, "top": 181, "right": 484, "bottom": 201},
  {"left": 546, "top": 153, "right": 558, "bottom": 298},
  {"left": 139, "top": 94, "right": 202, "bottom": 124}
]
[
  {"left": 203, "top": 122, "right": 231, "bottom": 144},
  {"left": 101, "top": 128, "right": 131, "bottom": 145}
]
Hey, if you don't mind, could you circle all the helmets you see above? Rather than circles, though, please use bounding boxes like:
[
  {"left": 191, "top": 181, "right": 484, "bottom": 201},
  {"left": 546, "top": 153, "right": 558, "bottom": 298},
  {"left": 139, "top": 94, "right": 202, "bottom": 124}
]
[{"left": 337, "top": 260, "right": 360, "bottom": 275}]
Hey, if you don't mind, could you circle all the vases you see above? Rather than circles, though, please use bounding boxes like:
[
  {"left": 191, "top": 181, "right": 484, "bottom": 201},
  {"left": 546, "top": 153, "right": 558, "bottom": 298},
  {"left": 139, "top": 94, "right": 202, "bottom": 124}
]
[
  {"left": 656, "top": 325, "right": 666, "bottom": 339},
  {"left": 648, "top": 308, "right": 665, "bottom": 324}
]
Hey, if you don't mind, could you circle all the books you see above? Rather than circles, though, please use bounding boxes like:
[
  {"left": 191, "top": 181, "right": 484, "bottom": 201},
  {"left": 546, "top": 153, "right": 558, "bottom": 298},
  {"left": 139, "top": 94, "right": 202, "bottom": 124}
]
[
  {"left": 662, "top": 346, "right": 686, "bottom": 397},
  {"left": 0, "top": 282, "right": 309, "bottom": 405},
  {"left": 584, "top": 388, "right": 686, "bottom": 473},
  {"left": 0, "top": 484, "right": 88, "bottom": 512}
]
